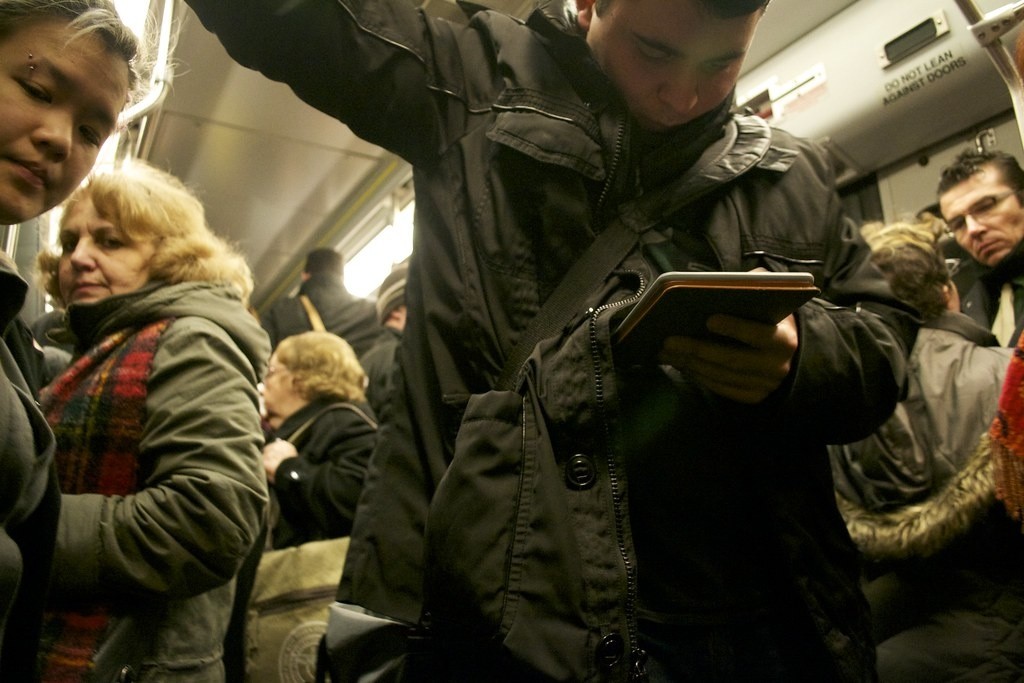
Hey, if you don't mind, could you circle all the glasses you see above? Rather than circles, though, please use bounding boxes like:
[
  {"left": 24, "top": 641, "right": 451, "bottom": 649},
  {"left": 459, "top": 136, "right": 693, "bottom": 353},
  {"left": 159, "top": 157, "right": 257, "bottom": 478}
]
[{"left": 944, "top": 190, "right": 1018, "bottom": 239}]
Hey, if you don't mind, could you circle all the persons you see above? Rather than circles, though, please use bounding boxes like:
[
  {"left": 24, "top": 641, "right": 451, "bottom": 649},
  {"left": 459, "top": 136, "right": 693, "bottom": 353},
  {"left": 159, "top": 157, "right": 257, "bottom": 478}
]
[
  {"left": 937, "top": 150, "right": 1024, "bottom": 356},
  {"left": 22, "top": 159, "right": 265, "bottom": 683},
  {"left": 222, "top": 333, "right": 386, "bottom": 683},
  {"left": 260, "top": 246, "right": 380, "bottom": 358},
  {"left": 827, "top": 221, "right": 1024, "bottom": 682},
  {"left": 187, "top": 0, "right": 909, "bottom": 683},
  {"left": 359, "top": 268, "right": 411, "bottom": 437},
  {"left": 0, "top": 0, "right": 175, "bottom": 683}
]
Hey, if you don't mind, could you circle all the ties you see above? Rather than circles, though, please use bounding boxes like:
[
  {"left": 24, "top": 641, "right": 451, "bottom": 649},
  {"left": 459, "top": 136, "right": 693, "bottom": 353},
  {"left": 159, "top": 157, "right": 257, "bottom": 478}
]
[{"left": 991, "top": 282, "right": 1016, "bottom": 348}]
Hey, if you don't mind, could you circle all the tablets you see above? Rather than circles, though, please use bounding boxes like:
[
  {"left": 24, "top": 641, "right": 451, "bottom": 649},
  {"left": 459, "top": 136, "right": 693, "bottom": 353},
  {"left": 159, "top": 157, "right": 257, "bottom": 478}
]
[{"left": 615, "top": 270, "right": 821, "bottom": 350}]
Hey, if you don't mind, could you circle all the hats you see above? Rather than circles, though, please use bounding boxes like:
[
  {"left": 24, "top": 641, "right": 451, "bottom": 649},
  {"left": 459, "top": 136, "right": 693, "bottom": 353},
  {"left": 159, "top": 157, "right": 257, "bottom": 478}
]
[{"left": 377, "top": 269, "right": 407, "bottom": 325}]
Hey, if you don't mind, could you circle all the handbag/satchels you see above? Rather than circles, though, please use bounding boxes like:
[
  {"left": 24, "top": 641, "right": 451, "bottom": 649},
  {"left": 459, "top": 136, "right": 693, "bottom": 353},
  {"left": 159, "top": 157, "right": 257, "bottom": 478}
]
[
  {"left": 317, "top": 602, "right": 433, "bottom": 683},
  {"left": 246, "top": 403, "right": 379, "bottom": 683}
]
